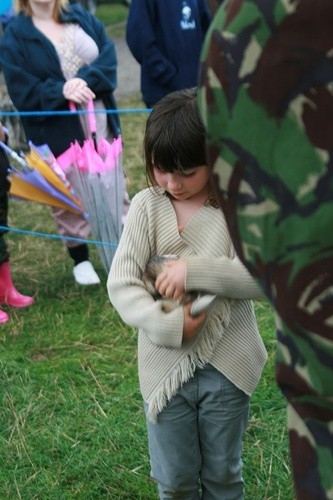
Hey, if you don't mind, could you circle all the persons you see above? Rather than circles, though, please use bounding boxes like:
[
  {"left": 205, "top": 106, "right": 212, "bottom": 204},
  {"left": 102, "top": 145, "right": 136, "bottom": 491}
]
[
  {"left": 197, "top": 0, "right": 333, "bottom": 500},
  {"left": 0, "top": 0, "right": 126, "bottom": 283},
  {"left": 106, "top": 87, "right": 268, "bottom": 500},
  {"left": 126, "top": 0, "right": 222, "bottom": 108},
  {"left": 0, "top": 124, "right": 34, "bottom": 324}
]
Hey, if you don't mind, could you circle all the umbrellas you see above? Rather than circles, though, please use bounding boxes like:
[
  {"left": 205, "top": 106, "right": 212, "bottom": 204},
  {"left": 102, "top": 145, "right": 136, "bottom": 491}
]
[
  {"left": 56, "top": 98, "right": 123, "bottom": 277},
  {"left": 0, "top": 127, "right": 87, "bottom": 219}
]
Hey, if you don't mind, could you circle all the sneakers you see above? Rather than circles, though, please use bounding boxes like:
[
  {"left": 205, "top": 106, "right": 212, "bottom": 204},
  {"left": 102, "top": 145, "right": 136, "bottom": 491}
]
[{"left": 73, "top": 261, "right": 100, "bottom": 285}]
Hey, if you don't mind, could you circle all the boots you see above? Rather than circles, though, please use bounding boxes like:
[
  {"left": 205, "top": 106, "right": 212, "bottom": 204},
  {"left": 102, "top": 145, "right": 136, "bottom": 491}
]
[{"left": 0, "top": 261, "right": 34, "bottom": 324}]
